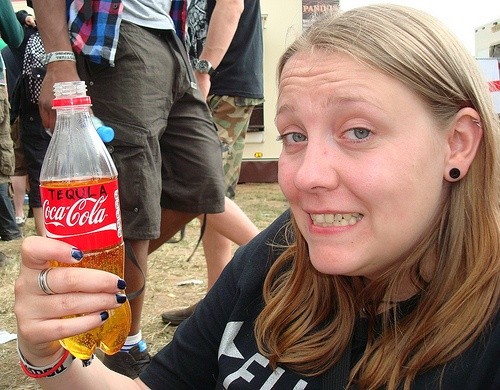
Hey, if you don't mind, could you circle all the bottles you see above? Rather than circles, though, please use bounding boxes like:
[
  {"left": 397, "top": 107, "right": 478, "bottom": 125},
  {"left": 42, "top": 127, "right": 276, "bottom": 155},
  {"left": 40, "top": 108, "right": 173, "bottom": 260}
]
[
  {"left": 40, "top": 79, "right": 132, "bottom": 362},
  {"left": 45, "top": 116, "right": 115, "bottom": 142}
]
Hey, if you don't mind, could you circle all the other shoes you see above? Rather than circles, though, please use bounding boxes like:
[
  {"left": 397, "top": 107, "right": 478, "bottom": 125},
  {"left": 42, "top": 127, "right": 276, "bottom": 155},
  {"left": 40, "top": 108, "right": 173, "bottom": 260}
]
[
  {"left": 16, "top": 216, "right": 26, "bottom": 227},
  {"left": 162, "top": 299, "right": 201, "bottom": 326},
  {"left": 2, "top": 230, "right": 20, "bottom": 242}
]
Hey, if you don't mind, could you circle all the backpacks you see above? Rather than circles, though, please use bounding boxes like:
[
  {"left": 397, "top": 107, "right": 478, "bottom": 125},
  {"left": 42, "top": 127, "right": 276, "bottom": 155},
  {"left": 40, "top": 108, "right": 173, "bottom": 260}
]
[{"left": 22, "top": 32, "right": 47, "bottom": 104}]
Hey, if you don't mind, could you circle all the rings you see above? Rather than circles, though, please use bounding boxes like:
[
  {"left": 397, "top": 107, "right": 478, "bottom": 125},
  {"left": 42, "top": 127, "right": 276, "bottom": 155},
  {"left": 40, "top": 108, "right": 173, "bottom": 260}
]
[{"left": 39, "top": 267, "right": 55, "bottom": 294}]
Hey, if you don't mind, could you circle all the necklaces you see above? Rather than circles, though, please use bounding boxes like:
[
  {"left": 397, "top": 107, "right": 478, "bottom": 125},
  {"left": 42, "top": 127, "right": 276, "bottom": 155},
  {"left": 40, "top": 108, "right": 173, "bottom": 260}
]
[{"left": 358, "top": 275, "right": 404, "bottom": 316}]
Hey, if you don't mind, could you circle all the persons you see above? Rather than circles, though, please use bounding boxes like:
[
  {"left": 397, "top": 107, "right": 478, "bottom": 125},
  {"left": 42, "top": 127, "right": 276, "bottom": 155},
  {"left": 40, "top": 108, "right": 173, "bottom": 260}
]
[
  {"left": 14, "top": 5, "right": 500, "bottom": 390},
  {"left": 0, "top": 0, "right": 264, "bottom": 380}
]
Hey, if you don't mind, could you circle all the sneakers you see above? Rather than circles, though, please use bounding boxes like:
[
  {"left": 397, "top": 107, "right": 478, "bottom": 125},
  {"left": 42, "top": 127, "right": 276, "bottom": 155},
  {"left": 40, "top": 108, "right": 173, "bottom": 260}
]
[{"left": 94, "top": 340, "right": 150, "bottom": 380}]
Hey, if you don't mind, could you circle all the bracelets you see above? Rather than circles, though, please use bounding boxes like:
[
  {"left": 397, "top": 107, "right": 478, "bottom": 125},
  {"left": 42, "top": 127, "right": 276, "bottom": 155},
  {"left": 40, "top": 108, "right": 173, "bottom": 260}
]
[
  {"left": 16, "top": 339, "right": 72, "bottom": 378},
  {"left": 20, "top": 351, "right": 70, "bottom": 379},
  {"left": 46, "top": 51, "right": 75, "bottom": 63}
]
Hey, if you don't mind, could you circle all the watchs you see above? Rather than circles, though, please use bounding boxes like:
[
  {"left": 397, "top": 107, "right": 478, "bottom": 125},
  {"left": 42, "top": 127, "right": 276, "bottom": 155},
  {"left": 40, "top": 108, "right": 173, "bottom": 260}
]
[{"left": 194, "top": 57, "right": 219, "bottom": 80}]
